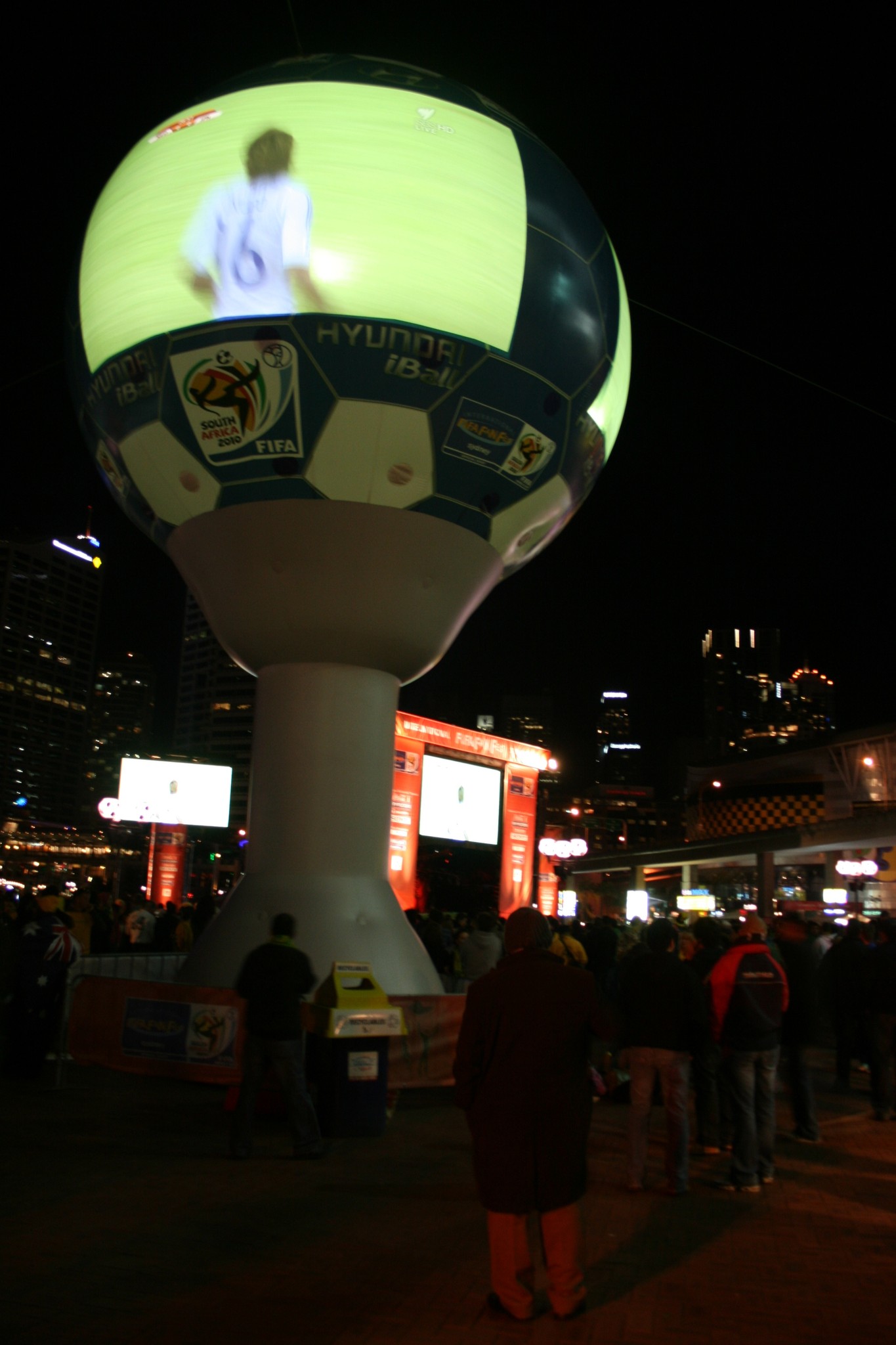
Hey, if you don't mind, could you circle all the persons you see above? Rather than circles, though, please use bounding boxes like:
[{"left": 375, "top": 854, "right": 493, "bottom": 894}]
[
  {"left": 450, "top": 908, "right": 595, "bottom": 1322},
  {"left": 408, "top": 904, "right": 506, "bottom": 995},
  {"left": 702, "top": 913, "right": 790, "bottom": 1193},
  {"left": 546, "top": 915, "right": 649, "bottom": 972},
  {"left": 615, "top": 919, "right": 697, "bottom": 1193},
  {"left": 0, "top": 880, "right": 218, "bottom": 1012},
  {"left": 675, "top": 916, "right": 742, "bottom": 981},
  {"left": 772, "top": 910, "right": 896, "bottom": 1144},
  {"left": 234, "top": 913, "right": 319, "bottom": 1159}
]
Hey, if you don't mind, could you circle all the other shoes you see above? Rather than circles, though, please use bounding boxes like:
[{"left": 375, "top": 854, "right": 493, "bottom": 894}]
[
  {"left": 874, "top": 1109, "right": 890, "bottom": 1122},
  {"left": 233, "top": 1147, "right": 250, "bottom": 1158},
  {"left": 717, "top": 1178, "right": 760, "bottom": 1194},
  {"left": 486, "top": 1291, "right": 534, "bottom": 1322},
  {"left": 786, "top": 1129, "right": 815, "bottom": 1144},
  {"left": 296, "top": 1149, "right": 328, "bottom": 1160},
  {"left": 689, "top": 1145, "right": 720, "bottom": 1155},
  {"left": 720, "top": 1143, "right": 733, "bottom": 1150},
  {"left": 762, "top": 1175, "right": 774, "bottom": 1185}
]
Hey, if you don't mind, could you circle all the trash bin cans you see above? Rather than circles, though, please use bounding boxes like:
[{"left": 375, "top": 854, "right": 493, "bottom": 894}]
[{"left": 302, "top": 962, "right": 411, "bottom": 1138}]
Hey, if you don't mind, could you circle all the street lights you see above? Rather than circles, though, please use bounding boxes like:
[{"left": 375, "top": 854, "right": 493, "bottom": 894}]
[
  {"left": 835, "top": 859, "right": 879, "bottom": 915},
  {"left": 538, "top": 837, "right": 588, "bottom": 925},
  {"left": 97, "top": 797, "right": 136, "bottom": 895}
]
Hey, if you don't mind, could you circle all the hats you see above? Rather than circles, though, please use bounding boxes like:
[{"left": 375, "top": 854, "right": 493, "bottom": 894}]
[{"left": 502, "top": 907, "right": 553, "bottom": 954}]
